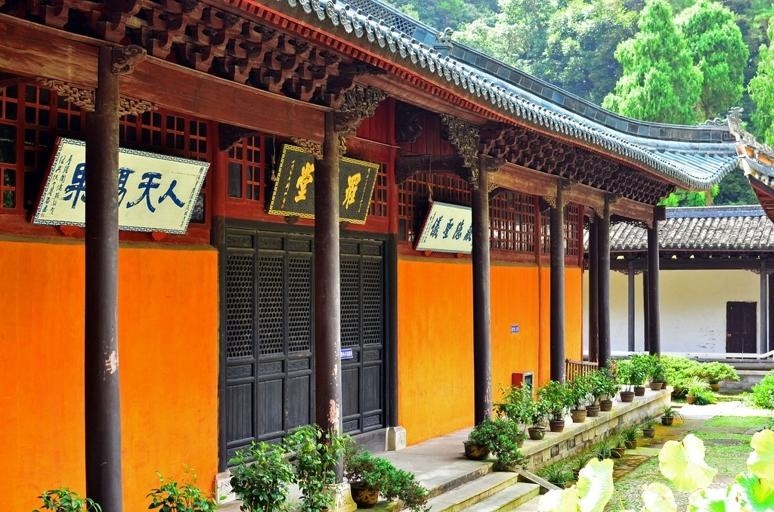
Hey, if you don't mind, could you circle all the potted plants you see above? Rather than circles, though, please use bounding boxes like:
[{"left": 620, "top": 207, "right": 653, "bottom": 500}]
[
  {"left": 282, "top": 424, "right": 347, "bottom": 512},
  {"left": 343, "top": 436, "right": 432, "bottom": 512},
  {"left": 463, "top": 354, "right": 741, "bottom": 488}
]
